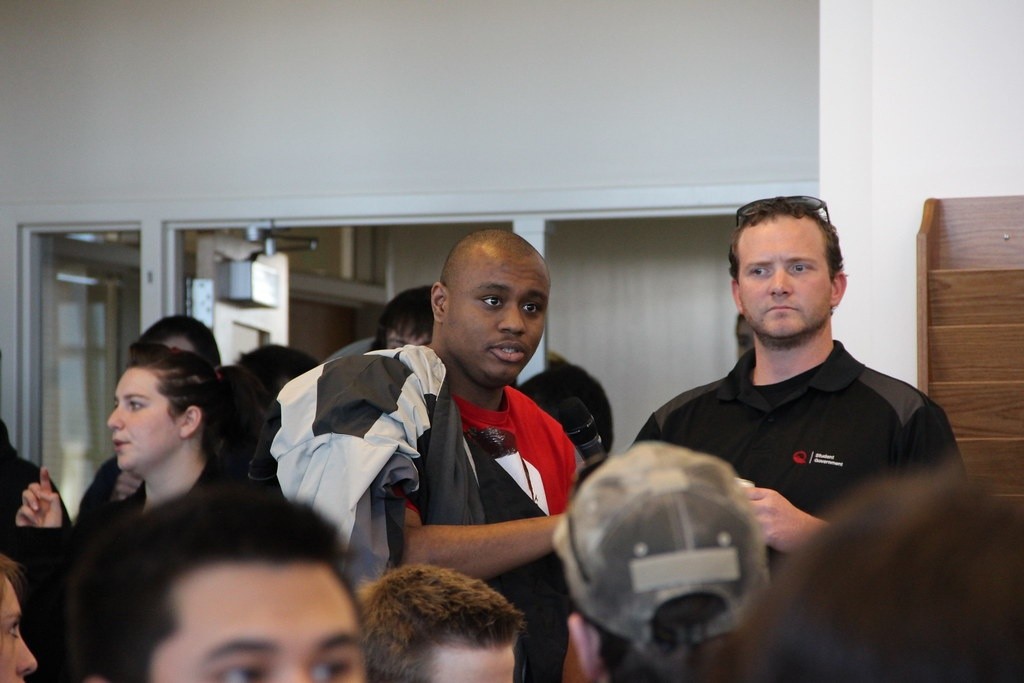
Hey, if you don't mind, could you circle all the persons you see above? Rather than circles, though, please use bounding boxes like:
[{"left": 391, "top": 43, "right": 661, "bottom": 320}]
[
  {"left": 0, "top": 552, "right": 38, "bottom": 683},
  {"left": 550, "top": 440, "right": 772, "bottom": 683},
  {"left": 0, "top": 313, "right": 612, "bottom": 683},
  {"left": 382, "top": 227, "right": 591, "bottom": 683},
  {"left": 75, "top": 488, "right": 370, "bottom": 683},
  {"left": 351, "top": 563, "right": 528, "bottom": 683},
  {"left": 571, "top": 195, "right": 964, "bottom": 683},
  {"left": 678, "top": 465, "right": 1024, "bottom": 683}
]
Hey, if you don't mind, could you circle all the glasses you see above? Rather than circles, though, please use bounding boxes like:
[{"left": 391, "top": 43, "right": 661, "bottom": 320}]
[{"left": 736, "top": 196, "right": 830, "bottom": 227}]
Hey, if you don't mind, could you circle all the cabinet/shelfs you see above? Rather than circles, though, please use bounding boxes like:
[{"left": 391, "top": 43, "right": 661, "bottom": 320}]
[{"left": 916, "top": 193, "right": 1024, "bottom": 510}]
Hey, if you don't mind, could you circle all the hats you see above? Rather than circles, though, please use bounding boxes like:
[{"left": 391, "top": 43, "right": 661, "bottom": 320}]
[{"left": 551, "top": 441, "right": 770, "bottom": 643}]
